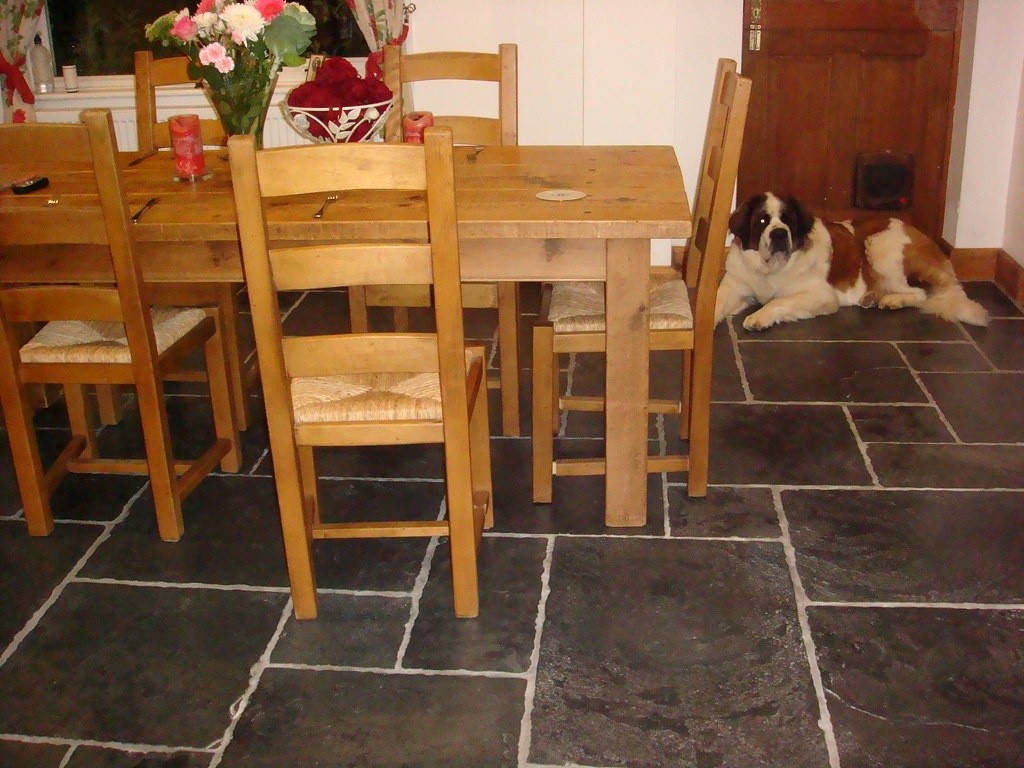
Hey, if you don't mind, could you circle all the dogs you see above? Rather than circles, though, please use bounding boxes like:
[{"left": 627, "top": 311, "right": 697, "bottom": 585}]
[{"left": 714, "top": 191, "right": 986, "bottom": 332}]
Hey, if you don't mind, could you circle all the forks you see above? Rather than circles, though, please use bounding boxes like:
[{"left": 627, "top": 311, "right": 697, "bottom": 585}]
[
  {"left": 466, "top": 148, "right": 484, "bottom": 161},
  {"left": 313, "top": 195, "right": 339, "bottom": 218}
]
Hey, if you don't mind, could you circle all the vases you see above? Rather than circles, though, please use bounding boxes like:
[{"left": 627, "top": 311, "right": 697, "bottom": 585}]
[
  {"left": 0, "top": 88, "right": 48, "bottom": 195},
  {"left": 203, "top": 74, "right": 279, "bottom": 159}
]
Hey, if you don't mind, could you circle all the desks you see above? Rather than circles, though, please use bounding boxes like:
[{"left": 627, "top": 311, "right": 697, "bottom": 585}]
[{"left": 0, "top": 145, "right": 693, "bottom": 526}]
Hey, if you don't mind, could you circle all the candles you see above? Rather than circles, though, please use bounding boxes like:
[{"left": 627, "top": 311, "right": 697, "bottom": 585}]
[
  {"left": 168, "top": 114, "right": 204, "bottom": 179},
  {"left": 403, "top": 111, "right": 433, "bottom": 145}
]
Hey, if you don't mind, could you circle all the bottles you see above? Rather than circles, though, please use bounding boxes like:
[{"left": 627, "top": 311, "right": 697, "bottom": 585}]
[{"left": 30, "top": 34, "right": 55, "bottom": 94}]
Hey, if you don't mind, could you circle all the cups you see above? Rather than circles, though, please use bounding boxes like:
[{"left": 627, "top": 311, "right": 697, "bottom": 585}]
[{"left": 62, "top": 65, "right": 80, "bottom": 92}]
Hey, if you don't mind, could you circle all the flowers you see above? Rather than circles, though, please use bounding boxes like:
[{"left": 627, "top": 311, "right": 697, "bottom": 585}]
[
  {"left": 0, "top": 0, "right": 44, "bottom": 108},
  {"left": 145, "top": 0, "right": 317, "bottom": 151}
]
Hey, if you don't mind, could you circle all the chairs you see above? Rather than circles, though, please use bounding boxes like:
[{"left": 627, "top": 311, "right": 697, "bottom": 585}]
[{"left": 0, "top": 44, "right": 752, "bottom": 620}]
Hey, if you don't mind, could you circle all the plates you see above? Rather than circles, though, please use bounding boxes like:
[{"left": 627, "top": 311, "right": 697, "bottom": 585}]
[{"left": 536, "top": 190, "right": 587, "bottom": 201}]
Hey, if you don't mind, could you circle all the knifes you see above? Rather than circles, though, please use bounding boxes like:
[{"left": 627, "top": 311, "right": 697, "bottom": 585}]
[
  {"left": 129, "top": 195, "right": 163, "bottom": 223},
  {"left": 127, "top": 152, "right": 158, "bottom": 166}
]
